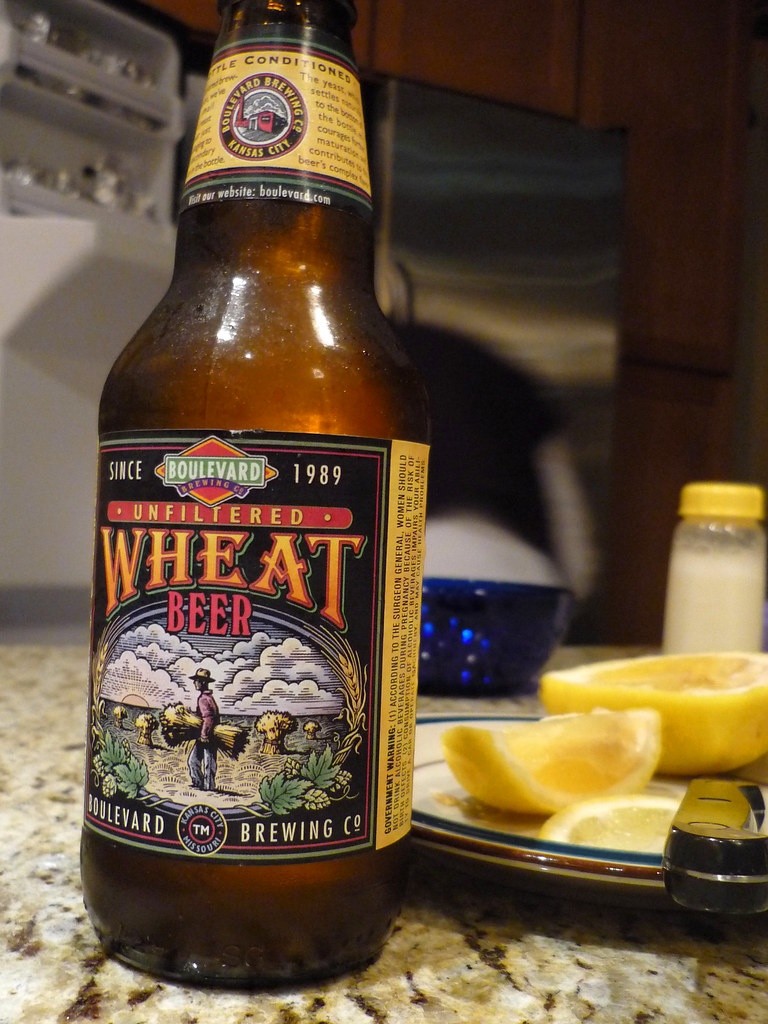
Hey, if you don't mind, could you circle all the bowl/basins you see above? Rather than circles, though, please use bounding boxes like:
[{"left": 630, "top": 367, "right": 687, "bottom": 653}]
[{"left": 411, "top": 577, "right": 577, "bottom": 699}]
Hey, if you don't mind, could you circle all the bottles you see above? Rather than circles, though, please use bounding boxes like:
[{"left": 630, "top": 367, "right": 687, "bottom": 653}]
[
  {"left": 405, "top": 717, "right": 768, "bottom": 910},
  {"left": 79, "top": 0, "right": 434, "bottom": 988},
  {"left": 666, "top": 481, "right": 768, "bottom": 661}
]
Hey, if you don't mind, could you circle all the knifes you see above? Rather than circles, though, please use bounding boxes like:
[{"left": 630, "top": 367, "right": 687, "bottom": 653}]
[{"left": 660, "top": 760, "right": 768, "bottom": 913}]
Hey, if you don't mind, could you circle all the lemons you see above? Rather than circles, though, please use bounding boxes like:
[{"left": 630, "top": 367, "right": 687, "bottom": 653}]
[
  {"left": 441, "top": 705, "right": 660, "bottom": 815},
  {"left": 536, "top": 793, "right": 681, "bottom": 853},
  {"left": 539, "top": 649, "right": 768, "bottom": 776}
]
